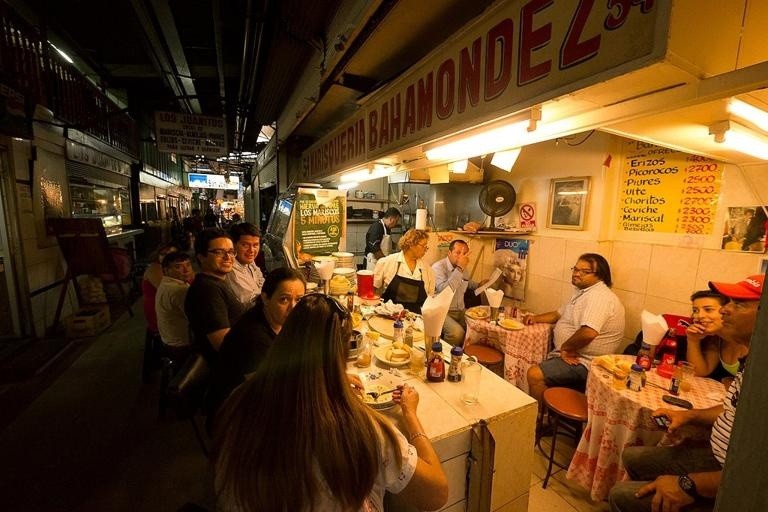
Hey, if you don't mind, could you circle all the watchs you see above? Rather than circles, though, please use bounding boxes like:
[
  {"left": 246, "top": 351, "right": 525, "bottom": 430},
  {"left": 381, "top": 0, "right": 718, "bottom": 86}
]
[{"left": 678, "top": 473, "right": 701, "bottom": 502}]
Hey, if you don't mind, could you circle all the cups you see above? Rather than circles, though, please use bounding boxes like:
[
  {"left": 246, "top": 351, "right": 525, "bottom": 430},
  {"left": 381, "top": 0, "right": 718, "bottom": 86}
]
[
  {"left": 356, "top": 269, "right": 375, "bottom": 299},
  {"left": 338, "top": 291, "right": 361, "bottom": 315},
  {"left": 357, "top": 334, "right": 373, "bottom": 367},
  {"left": 640, "top": 341, "right": 657, "bottom": 369},
  {"left": 680, "top": 361, "right": 696, "bottom": 392}
]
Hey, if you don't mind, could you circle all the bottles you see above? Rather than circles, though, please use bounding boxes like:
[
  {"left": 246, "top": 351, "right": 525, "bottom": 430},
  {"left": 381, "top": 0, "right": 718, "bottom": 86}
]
[
  {"left": 404, "top": 327, "right": 413, "bottom": 348},
  {"left": 494, "top": 301, "right": 519, "bottom": 322},
  {"left": 448, "top": 346, "right": 462, "bottom": 381},
  {"left": 460, "top": 363, "right": 481, "bottom": 405},
  {"left": 355, "top": 189, "right": 376, "bottom": 199},
  {"left": 626, "top": 327, "right": 684, "bottom": 394},
  {"left": 415, "top": 199, "right": 428, "bottom": 232},
  {"left": 372, "top": 210, "right": 379, "bottom": 219},
  {"left": 393, "top": 320, "right": 404, "bottom": 345},
  {"left": 426, "top": 342, "right": 445, "bottom": 381}
]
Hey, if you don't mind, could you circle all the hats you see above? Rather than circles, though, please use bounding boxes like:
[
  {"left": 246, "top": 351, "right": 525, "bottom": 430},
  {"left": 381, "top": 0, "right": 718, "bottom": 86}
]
[{"left": 708, "top": 275, "right": 765, "bottom": 300}]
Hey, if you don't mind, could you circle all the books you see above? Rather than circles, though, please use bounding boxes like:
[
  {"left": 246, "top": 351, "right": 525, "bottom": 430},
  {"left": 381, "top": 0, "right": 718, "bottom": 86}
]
[{"left": 472, "top": 268, "right": 503, "bottom": 296}]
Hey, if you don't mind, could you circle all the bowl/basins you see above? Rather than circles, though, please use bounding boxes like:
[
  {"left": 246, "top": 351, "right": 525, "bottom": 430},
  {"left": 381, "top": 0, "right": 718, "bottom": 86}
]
[{"left": 467, "top": 308, "right": 489, "bottom": 319}]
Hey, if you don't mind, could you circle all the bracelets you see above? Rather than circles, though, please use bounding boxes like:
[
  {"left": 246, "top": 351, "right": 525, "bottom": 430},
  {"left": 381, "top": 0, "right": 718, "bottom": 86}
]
[
  {"left": 410, "top": 432, "right": 429, "bottom": 442},
  {"left": 455, "top": 265, "right": 463, "bottom": 272}
]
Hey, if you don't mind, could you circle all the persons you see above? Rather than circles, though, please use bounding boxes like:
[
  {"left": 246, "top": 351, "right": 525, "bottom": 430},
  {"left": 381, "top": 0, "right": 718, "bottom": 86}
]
[
  {"left": 372, "top": 228, "right": 437, "bottom": 318},
  {"left": 524, "top": 254, "right": 626, "bottom": 435},
  {"left": 724, "top": 208, "right": 758, "bottom": 249},
  {"left": 212, "top": 293, "right": 449, "bottom": 511},
  {"left": 430, "top": 239, "right": 492, "bottom": 347},
  {"left": 363, "top": 207, "right": 401, "bottom": 272},
  {"left": 684, "top": 288, "right": 751, "bottom": 394},
  {"left": 141, "top": 207, "right": 306, "bottom": 410},
  {"left": 493, "top": 248, "right": 525, "bottom": 297},
  {"left": 610, "top": 273, "right": 767, "bottom": 511}
]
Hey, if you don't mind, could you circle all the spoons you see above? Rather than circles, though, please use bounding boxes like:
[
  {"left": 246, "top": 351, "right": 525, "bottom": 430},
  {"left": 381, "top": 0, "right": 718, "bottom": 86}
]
[{"left": 369, "top": 389, "right": 399, "bottom": 399}]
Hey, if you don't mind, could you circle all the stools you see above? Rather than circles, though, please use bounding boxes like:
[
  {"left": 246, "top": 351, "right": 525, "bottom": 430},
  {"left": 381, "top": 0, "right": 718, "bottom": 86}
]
[{"left": 464, "top": 343, "right": 588, "bottom": 488}]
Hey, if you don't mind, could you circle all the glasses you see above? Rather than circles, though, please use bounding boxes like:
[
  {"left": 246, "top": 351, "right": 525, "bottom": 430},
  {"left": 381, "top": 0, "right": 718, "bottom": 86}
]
[
  {"left": 571, "top": 268, "right": 597, "bottom": 274},
  {"left": 418, "top": 243, "right": 428, "bottom": 249},
  {"left": 208, "top": 249, "right": 238, "bottom": 257},
  {"left": 302, "top": 293, "right": 345, "bottom": 323}
]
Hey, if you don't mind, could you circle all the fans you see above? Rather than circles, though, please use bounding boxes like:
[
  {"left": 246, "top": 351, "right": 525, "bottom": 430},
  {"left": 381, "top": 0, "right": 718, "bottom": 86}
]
[{"left": 476, "top": 179, "right": 517, "bottom": 234}]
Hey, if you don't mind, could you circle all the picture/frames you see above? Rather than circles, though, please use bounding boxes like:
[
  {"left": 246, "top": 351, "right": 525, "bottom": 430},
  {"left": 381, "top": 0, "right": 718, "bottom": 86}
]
[{"left": 545, "top": 176, "right": 588, "bottom": 229}]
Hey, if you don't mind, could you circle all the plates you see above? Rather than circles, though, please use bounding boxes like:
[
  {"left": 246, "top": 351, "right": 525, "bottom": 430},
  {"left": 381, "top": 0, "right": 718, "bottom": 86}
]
[
  {"left": 353, "top": 371, "right": 404, "bottom": 404},
  {"left": 305, "top": 251, "right": 355, "bottom": 293},
  {"left": 499, "top": 319, "right": 523, "bottom": 330},
  {"left": 376, "top": 345, "right": 408, "bottom": 367}
]
